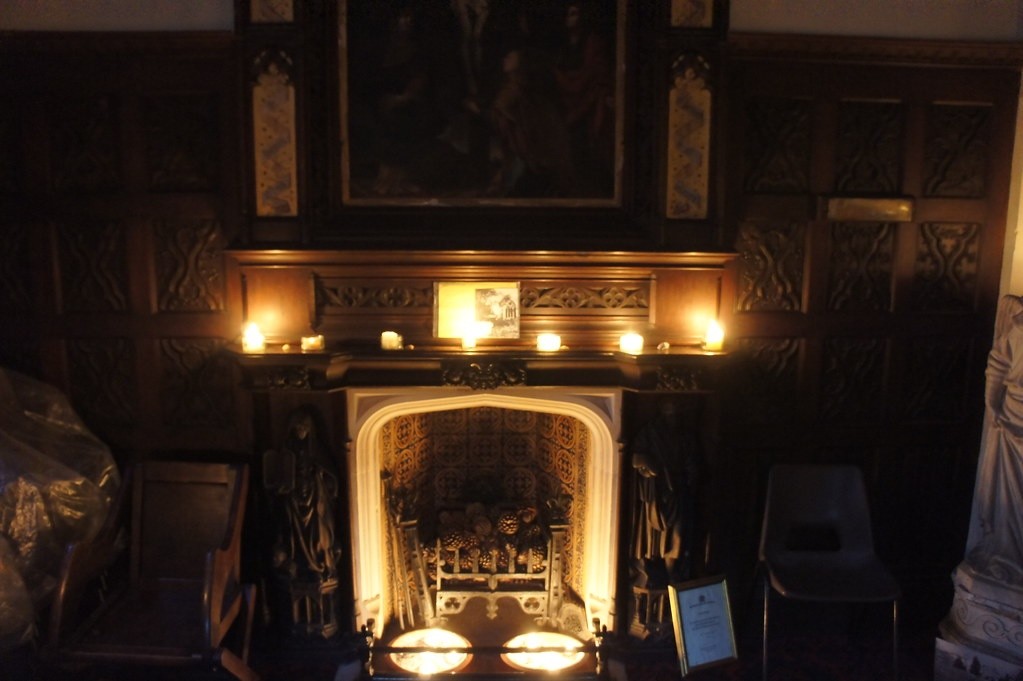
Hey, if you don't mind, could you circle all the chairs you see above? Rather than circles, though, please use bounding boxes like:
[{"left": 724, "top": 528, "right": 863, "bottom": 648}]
[
  {"left": 736, "top": 462, "right": 903, "bottom": 681},
  {"left": 44, "top": 453, "right": 264, "bottom": 681}
]
